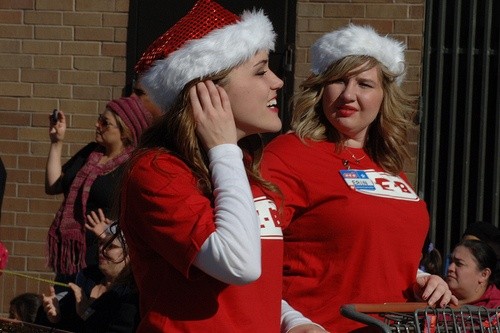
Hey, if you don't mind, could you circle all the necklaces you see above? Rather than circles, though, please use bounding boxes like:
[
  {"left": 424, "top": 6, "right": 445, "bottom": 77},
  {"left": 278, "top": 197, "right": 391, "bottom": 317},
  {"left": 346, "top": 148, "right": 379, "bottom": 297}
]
[{"left": 335, "top": 136, "right": 370, "bottom": 164}]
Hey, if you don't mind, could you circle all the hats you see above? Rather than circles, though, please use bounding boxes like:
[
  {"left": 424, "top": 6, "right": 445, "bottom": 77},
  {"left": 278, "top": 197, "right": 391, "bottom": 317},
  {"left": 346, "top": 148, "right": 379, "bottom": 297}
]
[
  {"left": 311, "top": 23, "right": 407, "bottom": 86},
  {"left": 133, "top": 0, "right": 277, "bottom": 113},
  {"left": 105, "top": 92, "right": 154, "bottom": 147}
]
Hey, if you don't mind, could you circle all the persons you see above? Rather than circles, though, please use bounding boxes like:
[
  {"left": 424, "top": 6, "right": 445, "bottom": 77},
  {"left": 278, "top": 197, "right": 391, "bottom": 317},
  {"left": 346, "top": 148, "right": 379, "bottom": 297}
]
[
  {"left": 425, "top": 221, "right": 500, "bottom": 333},
  {"left": 245, "top": 24, "right": 460, "bottom": 333},
  {"left": 109, "top": 0, "right": 329, "bottom": 333},
  {"left": 1, "top": 208, "right": 136, "bottom": 333},
  {"left": 44, "top": 92, "right": 164, "bottom": 298}
]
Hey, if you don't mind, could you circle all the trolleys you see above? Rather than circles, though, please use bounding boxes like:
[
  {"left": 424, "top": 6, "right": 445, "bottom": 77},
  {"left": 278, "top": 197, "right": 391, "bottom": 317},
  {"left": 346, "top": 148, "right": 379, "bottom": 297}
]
[{"left": 339, "top": 300, "right": 500, "bottom": 333}]
